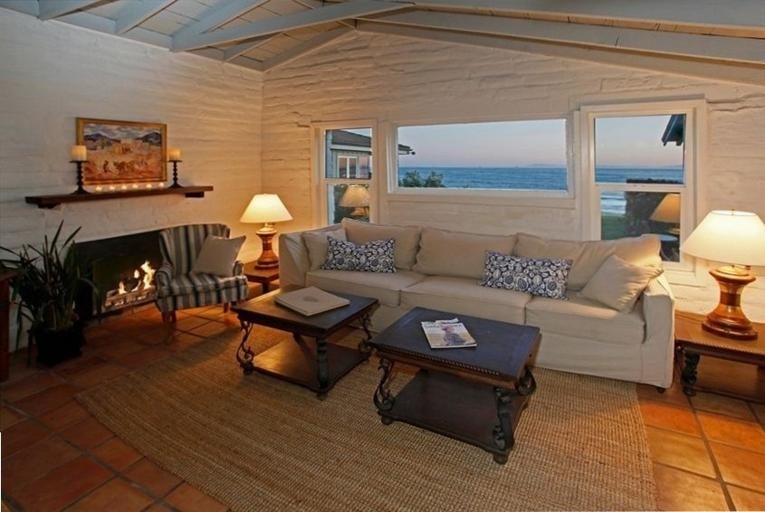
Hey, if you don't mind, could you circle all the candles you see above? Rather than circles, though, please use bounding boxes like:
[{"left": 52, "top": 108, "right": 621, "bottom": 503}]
[
  {"left": 71, "top": 145, "right": 87, "bottom": 161},
  {"left": 168, "top": 148, "right": 180, "bottom": 161}
]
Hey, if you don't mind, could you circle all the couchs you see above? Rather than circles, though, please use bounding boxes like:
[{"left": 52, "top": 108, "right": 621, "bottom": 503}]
[{"left": 281, "top": 217, "right": 675, "bottom": 392}]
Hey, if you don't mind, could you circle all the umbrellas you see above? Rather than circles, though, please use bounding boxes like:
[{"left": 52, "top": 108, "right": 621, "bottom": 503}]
[{"left": 661, "top": 114, "right": 683, "bottom": 146}]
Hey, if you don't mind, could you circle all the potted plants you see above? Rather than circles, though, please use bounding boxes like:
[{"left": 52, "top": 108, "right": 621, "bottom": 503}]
[{"left": 0, "top": 219, "right": 100, "bottom": 367}]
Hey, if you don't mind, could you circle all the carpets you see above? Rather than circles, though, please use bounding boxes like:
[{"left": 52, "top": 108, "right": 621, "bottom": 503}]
[{"left": 71, "top": 323, "right": 657, "bottom": 512}]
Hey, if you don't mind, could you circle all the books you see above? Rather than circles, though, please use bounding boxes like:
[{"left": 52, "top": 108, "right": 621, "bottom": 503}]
[
  {"left": 272, "top": 285, "right": 349, "bottom": 317},
  {"left": 420, "top": 318, "right": 478, "bottom": 349}
]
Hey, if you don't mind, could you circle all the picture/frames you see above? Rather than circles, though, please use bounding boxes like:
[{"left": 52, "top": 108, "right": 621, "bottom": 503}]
[{"left": 76, "top": 118, "right": 167, "bottom": 185}]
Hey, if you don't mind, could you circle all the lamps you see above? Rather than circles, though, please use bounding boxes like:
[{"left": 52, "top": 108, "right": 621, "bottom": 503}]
[
  {"left": 679, "top": 209, "right": 765, "bottom": 340},
  {"left": 648, "top": 194, "right": 681, "bottom": 236},
  {"left": 240, "top": 194, "right": 294, "bottom": 270},
  {"left": 338, "top": 185, "right": 371, "bottom": 219}
]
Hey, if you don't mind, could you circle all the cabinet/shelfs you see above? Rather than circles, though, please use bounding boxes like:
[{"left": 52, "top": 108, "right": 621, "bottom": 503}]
[{"left": 0, "top": 270, "right": 24, "bottom": 381}]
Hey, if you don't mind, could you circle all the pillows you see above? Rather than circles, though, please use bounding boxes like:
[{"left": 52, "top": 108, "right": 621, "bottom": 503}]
[
  {"left": 479, "top": 249, "right": 574, "bottom": 301},
  {"left": 193, "top": 233, "right": 245, "bottom": 277},
  {"left": 320, "top": 235, "right": 397, "bottom": 274},
  {"left": 301, "top": 228, "right": 349, "bottom": 272},
  {"left": 579, "top": 256, "right": 670, "bottom": 313}
]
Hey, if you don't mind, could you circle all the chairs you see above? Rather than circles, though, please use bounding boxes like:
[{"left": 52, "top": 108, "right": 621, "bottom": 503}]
[{"left": 154, "top": 224, "right": 254, "bottom": 345}]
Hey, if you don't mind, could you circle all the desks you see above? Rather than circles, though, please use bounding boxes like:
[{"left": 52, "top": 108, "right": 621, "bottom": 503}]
[
  {"left": 231, "top": 284, "right": 379, "bottom": 401},
  {"left": 642, "top": 232, "right": 679, "bottom": 262},
  {"left": 240, "top": 256, "right": 281, "bottom": 295},
  {"left": 367, "top": 305, "right": 541, "bottom": 464},
  {"left": 676, "top": 309, "right": 765, "bottom": 404}
]
